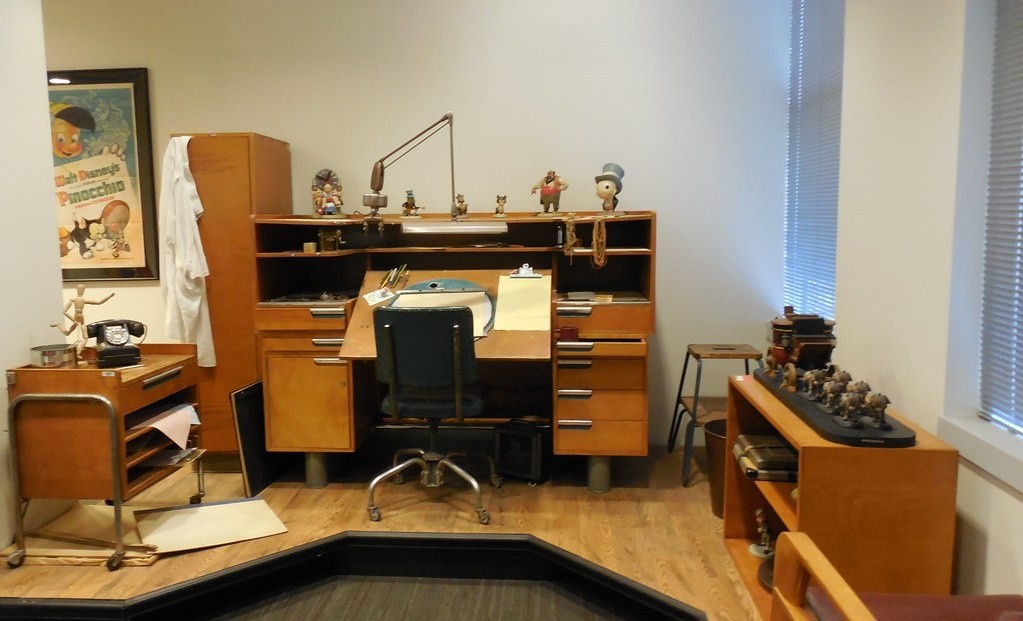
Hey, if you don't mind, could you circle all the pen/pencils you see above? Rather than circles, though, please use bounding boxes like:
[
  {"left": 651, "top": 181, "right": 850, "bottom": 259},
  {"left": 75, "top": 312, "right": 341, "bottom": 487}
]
[{"left": 377, "top": 264, "right": 407, "bottom": 288}]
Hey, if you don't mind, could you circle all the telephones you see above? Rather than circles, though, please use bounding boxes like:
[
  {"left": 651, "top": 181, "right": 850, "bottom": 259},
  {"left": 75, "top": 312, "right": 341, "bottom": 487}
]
[{"left": 87, "top": 319, "right": 148, "bottom": 369}]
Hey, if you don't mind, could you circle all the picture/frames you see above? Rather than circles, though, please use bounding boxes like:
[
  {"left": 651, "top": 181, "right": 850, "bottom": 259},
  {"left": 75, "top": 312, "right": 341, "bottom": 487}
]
[{"left": 47, "top": 67, "right": 161, "bottom": 281}]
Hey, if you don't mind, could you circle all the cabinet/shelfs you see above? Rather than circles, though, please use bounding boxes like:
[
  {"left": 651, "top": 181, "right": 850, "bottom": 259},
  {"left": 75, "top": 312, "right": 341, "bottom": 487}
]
[
  {"left": 166, "top": 132, "right": 658, "bottom": 495},
  {"left": 10, "top": 343, "right": 203, "bottom": 551},
  {"left": 720, "top": 372, "right": 958, "bottom": 621}
]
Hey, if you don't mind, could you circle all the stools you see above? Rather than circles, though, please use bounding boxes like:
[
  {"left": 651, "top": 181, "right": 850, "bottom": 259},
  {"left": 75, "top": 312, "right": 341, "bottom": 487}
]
[{"left": 667, "top": 343, "right": 768, "bottom": 485}]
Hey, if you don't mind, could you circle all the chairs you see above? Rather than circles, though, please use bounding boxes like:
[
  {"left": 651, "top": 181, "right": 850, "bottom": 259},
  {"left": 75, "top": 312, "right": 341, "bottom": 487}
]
[
  {"left": 366, "top": 305, "right": 505, "bottom": 527},
  {"left": 768, "top": 531, "right": 1022, "bottom": 621}
]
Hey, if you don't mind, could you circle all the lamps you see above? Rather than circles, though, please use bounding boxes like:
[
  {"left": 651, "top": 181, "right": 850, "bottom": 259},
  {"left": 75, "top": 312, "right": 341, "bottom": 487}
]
[{"left": 360, "top": 112, "right": 508, "bottom": 237}]
